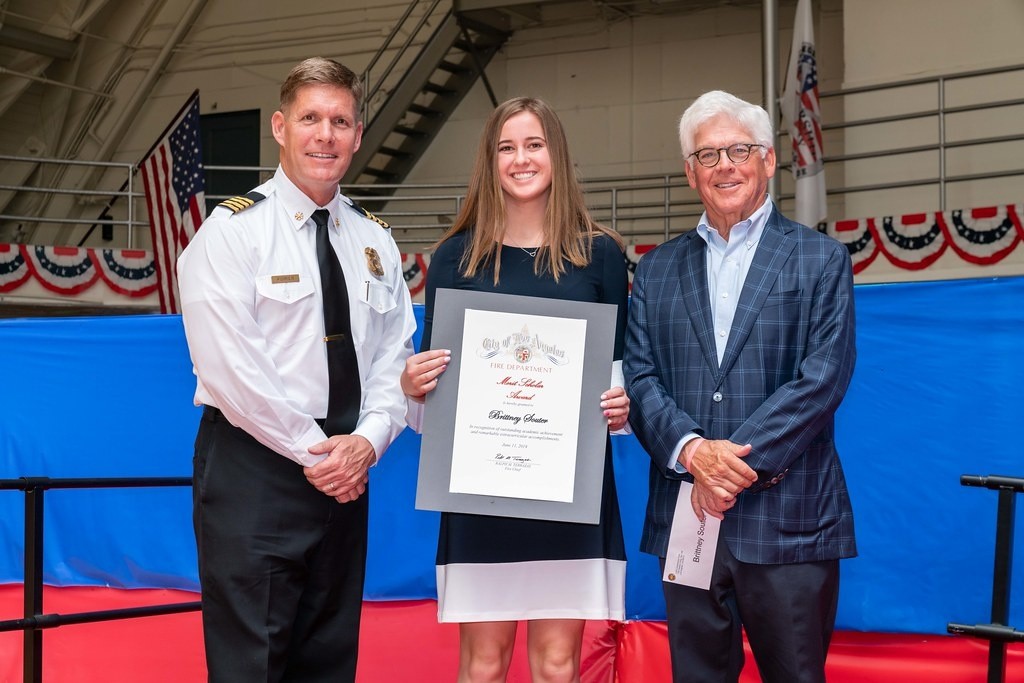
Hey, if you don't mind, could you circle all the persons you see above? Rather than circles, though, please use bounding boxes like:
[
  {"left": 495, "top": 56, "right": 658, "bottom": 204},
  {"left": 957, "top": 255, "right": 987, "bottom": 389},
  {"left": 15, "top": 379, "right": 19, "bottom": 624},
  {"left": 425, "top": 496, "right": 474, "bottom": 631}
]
[
  {"left": 623, "top": 89, "right": 858, "bottom": 683},
  {"left": 400, "top": 96, "right": 629, "bottom": 683},
  {"left": 175, "top": 58, "right": 417, "bottom": 683}
]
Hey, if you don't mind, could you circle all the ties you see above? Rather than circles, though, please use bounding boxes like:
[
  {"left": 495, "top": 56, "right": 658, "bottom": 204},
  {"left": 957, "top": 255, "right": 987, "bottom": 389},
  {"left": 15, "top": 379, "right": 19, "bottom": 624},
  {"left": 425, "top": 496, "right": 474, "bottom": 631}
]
[{"left": 310, "top": 209, "right": 361, "bottom": 438}]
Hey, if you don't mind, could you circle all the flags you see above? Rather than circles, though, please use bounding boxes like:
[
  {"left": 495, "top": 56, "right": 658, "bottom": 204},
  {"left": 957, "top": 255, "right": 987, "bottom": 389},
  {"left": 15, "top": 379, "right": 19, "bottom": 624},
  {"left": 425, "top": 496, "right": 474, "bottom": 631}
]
[
  {"left": 142, "top": 96, "right": 206, "bottom": 315},
  {"left": 778, "top": 0, "right": 827, "bottom": 229}
]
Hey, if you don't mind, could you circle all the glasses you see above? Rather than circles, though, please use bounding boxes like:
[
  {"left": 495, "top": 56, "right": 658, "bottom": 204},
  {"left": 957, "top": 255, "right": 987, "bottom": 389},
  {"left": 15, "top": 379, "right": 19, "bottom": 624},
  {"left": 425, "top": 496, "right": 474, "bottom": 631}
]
[{"left": 690, "top": 144, "right": 765, "bottom": 168}]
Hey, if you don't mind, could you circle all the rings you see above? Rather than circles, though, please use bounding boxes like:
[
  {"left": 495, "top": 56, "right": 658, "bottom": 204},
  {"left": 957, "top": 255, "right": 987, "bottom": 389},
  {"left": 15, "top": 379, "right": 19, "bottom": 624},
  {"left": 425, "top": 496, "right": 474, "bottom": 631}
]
[{"left": 329, "top": 482, "right": 335, "bottom": 491}]
